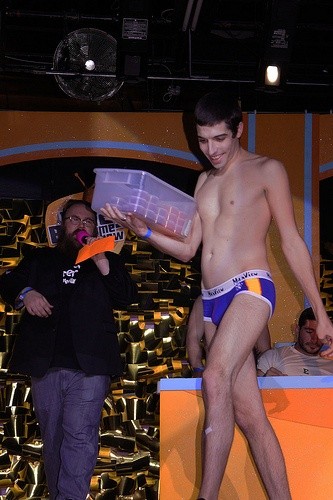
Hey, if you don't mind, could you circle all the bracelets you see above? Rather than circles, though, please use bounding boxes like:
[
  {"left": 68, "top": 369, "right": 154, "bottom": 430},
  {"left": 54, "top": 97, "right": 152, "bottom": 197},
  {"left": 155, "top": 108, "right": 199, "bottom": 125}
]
[
  {"left": 137, "top": 225, "right": 151, "bottom": 240},
  {"left": 19, "top": 287, "right": 31, "bottom": 300},
  {"left": 190, "top": 367, "right": 204, "bottom": 375}
]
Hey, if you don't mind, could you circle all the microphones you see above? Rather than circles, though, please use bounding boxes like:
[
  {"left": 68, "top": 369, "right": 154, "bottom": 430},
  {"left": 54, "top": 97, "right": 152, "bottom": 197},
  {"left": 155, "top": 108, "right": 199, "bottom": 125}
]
[{"left": 75, "top": 230, "right": 91, "bottom": 244}]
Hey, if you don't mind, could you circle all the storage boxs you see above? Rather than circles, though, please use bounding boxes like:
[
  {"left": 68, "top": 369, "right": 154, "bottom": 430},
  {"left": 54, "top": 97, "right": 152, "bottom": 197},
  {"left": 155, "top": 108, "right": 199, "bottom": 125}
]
[{"left": 91, "top": 168, "right": 196, "bottom": 242}]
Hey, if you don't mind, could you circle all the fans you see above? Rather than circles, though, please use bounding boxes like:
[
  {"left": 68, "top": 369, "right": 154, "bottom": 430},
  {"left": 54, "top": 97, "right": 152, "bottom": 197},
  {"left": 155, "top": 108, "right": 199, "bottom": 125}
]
[{"left": 52, "top": 27, "right": 123, "bottom": 103}]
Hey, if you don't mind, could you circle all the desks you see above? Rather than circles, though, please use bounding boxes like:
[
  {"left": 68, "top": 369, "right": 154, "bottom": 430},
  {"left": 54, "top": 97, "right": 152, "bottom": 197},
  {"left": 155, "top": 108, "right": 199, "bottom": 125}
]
[{"left": 158, "top": 376, "right": 333, "bottom": 500}]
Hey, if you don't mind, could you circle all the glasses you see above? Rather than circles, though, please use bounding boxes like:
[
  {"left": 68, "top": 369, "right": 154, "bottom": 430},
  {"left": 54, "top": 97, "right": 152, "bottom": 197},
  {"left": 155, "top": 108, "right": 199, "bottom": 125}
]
[{"left": 63, "top": 215, "right": 96, "bottom": 229}]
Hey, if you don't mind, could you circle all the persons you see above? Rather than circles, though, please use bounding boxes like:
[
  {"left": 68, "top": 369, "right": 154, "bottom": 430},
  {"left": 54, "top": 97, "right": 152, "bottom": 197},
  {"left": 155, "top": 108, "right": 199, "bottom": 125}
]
[
  {"left": 183, "top": 290, "right": 271, "bottom": 378},
  {"left": 0, "top": 198, "right": 137, "bottom": 500},
  {"left": 255, "top": 307, "right": 333, "bottom": 376},
  {"left": 103, "top": 90, "right": 333, "bottom": 500}
]
